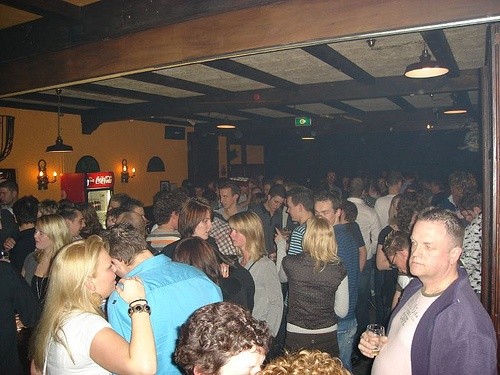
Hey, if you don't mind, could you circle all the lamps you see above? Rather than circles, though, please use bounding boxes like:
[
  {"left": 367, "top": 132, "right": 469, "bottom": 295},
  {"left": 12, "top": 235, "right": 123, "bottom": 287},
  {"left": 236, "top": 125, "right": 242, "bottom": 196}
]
[
  {"left": 121, "top": 160, "right": 136, "bottom": 183},
  {"left": 405, "top": 45, "right": 450, "bottom": 79},
  {"left": 443, "top": 94, "right": 467, "bottom": 114},
  {"left": 46, "top": 87, "right": 73, "bottom": 152},
  {"left": 217, "top": 117, "right": 236, "bottom": 129},
  {"left": 37, "top": 160, "right": 57, "bottom": 190}
]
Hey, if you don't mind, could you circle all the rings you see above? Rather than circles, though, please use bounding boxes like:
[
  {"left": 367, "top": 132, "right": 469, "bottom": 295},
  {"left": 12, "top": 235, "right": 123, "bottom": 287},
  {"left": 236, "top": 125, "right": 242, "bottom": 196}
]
[
  {"left": 221, "top": 269, "right": 224, "bottom": 272},
  {"left": 224, "top": 270, "right": 227, "bottom": 272}
]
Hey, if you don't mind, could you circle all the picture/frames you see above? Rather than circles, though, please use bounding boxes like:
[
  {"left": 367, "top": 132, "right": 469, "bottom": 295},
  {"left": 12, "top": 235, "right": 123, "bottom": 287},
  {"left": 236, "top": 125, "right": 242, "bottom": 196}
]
[{"left": 160, "top": 181, "right": 169, "bottom": 191}]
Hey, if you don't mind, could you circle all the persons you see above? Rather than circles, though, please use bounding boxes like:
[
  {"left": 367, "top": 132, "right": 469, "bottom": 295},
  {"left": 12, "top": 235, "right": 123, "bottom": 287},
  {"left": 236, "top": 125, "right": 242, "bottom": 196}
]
[
  {"left": 358, "top": 208, "right": 499, "bottom": 375},
  {"left": 26, "top": 234, "right": 157, "bottom": 375},
  {"left": 0, "top": 169, "right": 484, "bottom": 375}
]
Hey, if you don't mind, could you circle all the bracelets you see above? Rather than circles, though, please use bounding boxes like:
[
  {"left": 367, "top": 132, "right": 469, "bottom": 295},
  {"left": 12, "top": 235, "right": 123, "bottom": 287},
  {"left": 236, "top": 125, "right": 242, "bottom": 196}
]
[
  {"left": 128, "top": 303, "right": 151, "bottom": 318},
  {"left": 129, "top": 299, "right": 148, "bottom": 307}
]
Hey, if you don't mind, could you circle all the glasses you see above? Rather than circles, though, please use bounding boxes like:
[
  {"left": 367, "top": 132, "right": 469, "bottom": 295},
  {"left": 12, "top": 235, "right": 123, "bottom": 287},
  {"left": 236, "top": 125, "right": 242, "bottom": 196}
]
[{"left": 389, "top": 249, "right": 403, "bottom": 269}]
